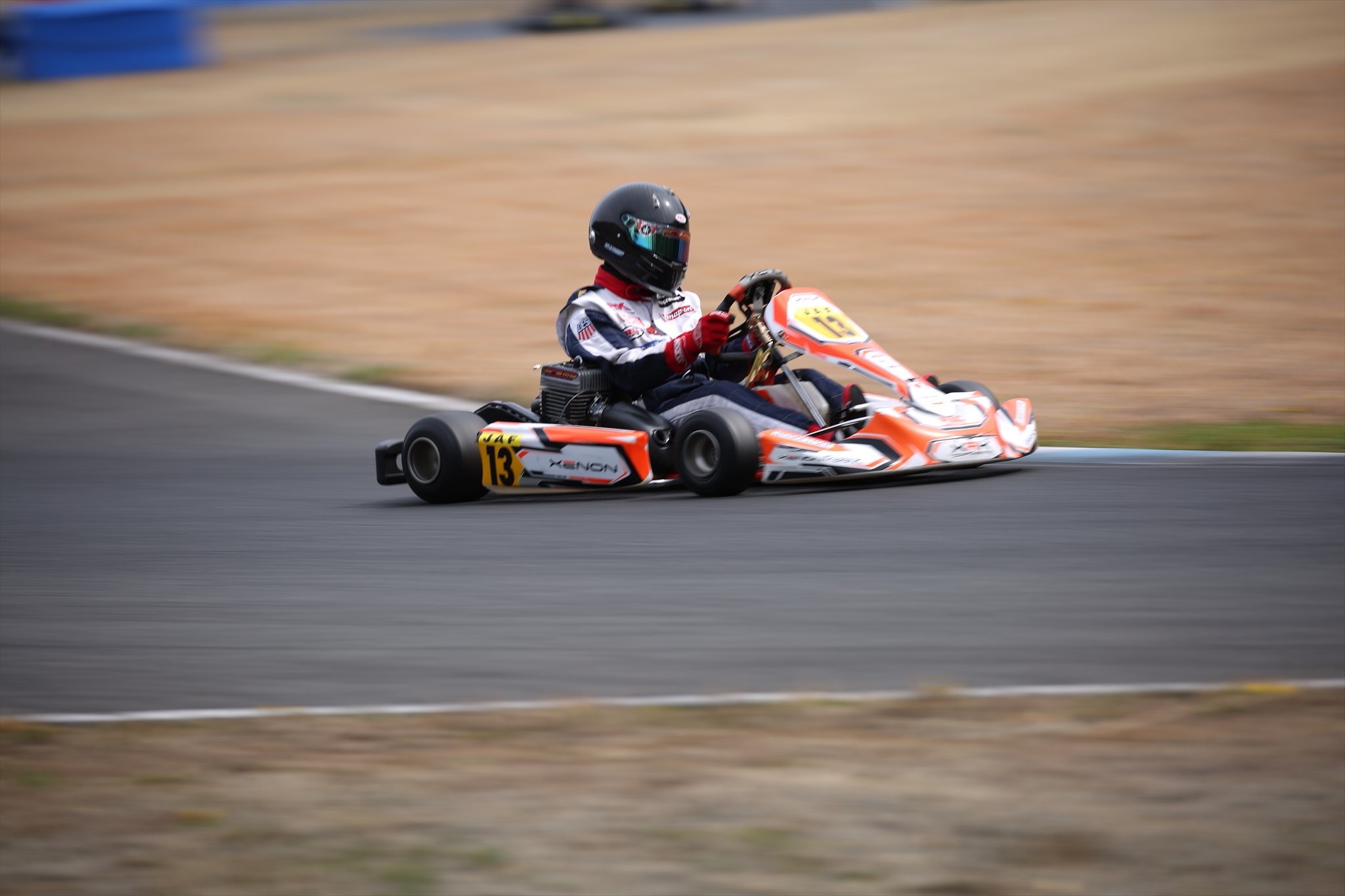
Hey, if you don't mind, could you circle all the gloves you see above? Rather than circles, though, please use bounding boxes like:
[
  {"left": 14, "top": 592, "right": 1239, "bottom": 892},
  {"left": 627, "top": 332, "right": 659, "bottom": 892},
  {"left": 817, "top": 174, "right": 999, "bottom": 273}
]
[
  {"left": 741, "top": 284, "right": 792, "bottom": 352},
  {"left": 664, "top": 310, "right": 735, "bottom": 373}
]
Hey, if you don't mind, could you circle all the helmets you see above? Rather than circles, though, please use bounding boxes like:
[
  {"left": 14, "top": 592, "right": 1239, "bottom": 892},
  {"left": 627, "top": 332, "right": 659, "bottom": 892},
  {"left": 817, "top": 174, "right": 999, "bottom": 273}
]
[{"left": 588, "top": 182, "right": 691, "bottom": 297}]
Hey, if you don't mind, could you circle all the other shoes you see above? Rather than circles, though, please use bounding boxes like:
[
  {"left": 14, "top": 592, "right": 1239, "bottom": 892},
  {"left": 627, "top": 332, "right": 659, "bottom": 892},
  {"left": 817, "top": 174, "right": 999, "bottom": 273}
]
[
  {"left": 830, "top": 383, "right": 868, "bottom": 442},
  {"left": 923, "top": 374, "right": 938, "bottom": 387}
]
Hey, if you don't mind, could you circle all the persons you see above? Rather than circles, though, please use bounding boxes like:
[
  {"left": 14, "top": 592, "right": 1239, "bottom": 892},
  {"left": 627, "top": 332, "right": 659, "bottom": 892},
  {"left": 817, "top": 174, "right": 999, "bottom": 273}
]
[{"left": 557, "top": 182, "right": 937, "bottom": 442}]
